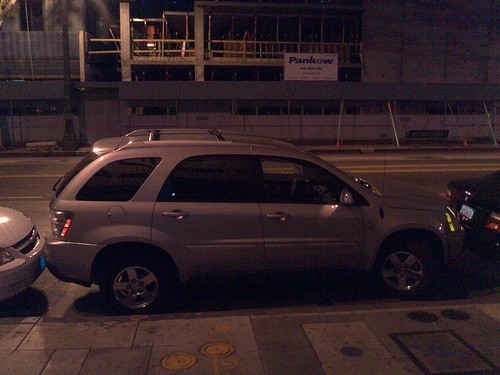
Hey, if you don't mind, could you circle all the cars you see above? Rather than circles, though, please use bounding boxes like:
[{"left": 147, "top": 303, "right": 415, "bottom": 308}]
[
  {"left": 0, "top": 205, "right": 47, "bottom": 302},
  {"left": 444, "top": 170, "right": 500, "bottom": 264}
]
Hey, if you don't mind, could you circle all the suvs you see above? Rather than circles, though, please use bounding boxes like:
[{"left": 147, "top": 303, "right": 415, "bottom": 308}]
[{"left": 46, "top": 128, "right": 467, "bottom": 316}]
